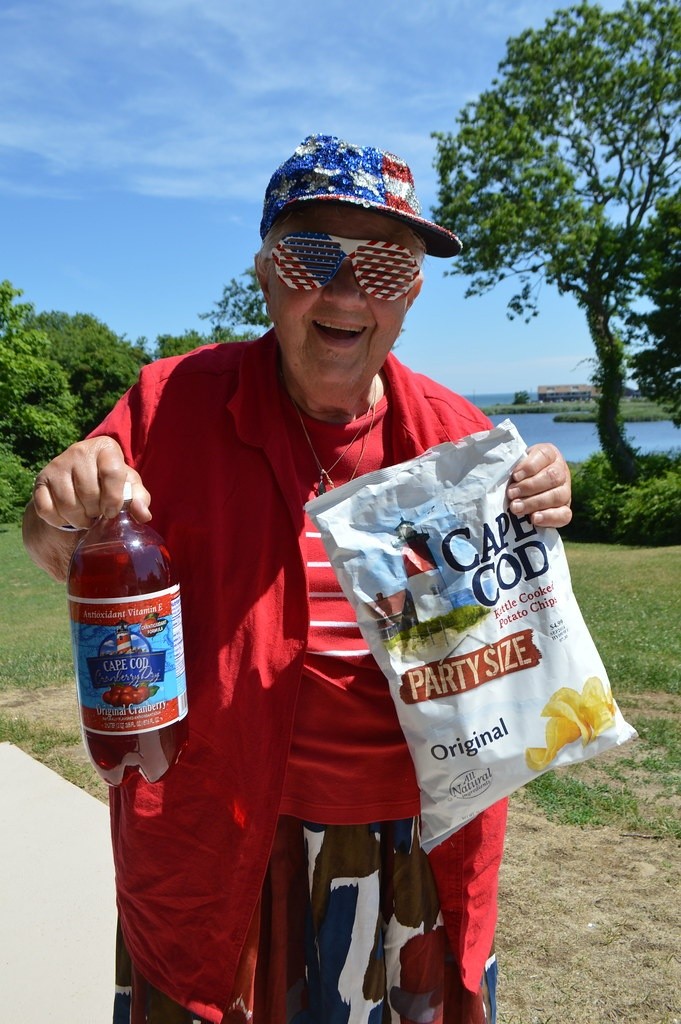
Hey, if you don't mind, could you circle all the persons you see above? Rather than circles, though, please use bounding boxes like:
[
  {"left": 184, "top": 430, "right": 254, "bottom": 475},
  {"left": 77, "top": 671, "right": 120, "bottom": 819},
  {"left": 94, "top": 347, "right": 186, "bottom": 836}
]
[{"left": 22, "top": 135, "right": 572, "bottom": 1024}]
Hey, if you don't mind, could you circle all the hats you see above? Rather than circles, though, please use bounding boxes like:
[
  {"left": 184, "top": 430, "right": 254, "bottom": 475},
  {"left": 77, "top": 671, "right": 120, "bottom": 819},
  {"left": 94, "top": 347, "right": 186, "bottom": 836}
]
[{"left": 259, "top": 134, "right": 464, "bottom": 259}]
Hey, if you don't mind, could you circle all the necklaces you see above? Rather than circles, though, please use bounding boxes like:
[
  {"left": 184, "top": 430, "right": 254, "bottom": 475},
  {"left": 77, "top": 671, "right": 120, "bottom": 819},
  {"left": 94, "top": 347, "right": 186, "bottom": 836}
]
[{"left": 292, "top": 381, "right": 379, "bottom": 499}]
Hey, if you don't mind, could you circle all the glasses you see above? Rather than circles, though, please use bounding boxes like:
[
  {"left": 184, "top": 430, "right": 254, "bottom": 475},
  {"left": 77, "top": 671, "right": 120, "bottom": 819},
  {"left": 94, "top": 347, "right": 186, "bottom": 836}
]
[{"left": 264, "top": 231, "right": 423, "bottom": 302}]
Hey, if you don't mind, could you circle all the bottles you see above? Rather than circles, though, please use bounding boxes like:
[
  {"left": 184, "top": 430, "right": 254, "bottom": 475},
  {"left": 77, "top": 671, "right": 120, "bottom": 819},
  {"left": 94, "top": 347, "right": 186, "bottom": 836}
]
[{"left": 66, "top": 482, "right": 190, "bottom": 785}]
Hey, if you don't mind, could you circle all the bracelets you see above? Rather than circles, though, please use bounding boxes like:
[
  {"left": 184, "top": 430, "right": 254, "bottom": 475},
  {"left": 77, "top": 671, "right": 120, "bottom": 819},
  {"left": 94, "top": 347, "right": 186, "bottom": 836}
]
[{"left": 58, "top": 525, "right": 84, "bottom": 532}]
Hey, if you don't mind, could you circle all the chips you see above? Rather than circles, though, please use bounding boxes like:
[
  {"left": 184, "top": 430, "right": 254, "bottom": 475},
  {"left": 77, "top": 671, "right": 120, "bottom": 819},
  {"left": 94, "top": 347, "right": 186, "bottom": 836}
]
[{"left": 523, "top": 676, "right": 619, "bottom": 771}]
